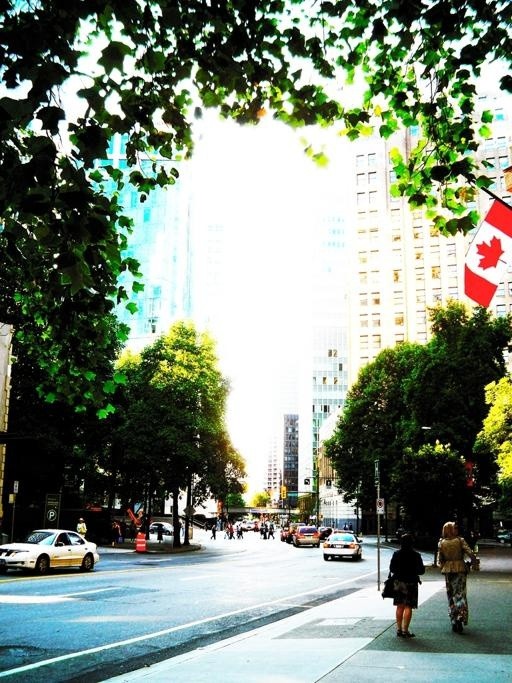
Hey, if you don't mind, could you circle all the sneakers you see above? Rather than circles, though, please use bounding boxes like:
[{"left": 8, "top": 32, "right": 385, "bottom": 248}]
[
  {"left": 396, "top": 629, "right": 415, "bottom": 637},
  {"left": 452, "top": 622, "right": 463, "bottom": 633}
]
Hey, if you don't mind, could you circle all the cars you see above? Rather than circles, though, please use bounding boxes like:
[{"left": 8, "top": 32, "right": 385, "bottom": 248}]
[
  {"left": 149, "top": 522, "right": 174, "bottom": 535},
  {"left": 280, "top": 523, "right": 334, "bottom": 547},
  {"left": 0, "top": 529, "right": 99, "bottom": 575},
  {"left": 497, "top": 530, "right": 512, "bottom": 544},
  {"left": 232, "top": 520, "right": 276, "bottom": 531},
  {"left": 323, "top": 530, "right": 362, "bottom": 561}
]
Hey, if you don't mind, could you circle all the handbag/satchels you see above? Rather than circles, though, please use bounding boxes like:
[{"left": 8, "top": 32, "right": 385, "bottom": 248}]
[
  {"left": 118, "top": 536, "right": 123, "bottom": 544},
  {"left": 381, "top": 572, "right": 395, "bottom": 600}
]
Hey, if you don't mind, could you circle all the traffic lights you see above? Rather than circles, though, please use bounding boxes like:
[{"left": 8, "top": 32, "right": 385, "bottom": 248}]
[
  {"left": 354, "top": 509, "right": 357, "bottom": 515},
  {"left": 304, "top": 479, "right": 310, "bottom": 485},
  {"left": 282, "top": 488, "right": 286, "bottom": 499},
  {"left": 325, "top": 480, "right": 332, "bottom": 486}
]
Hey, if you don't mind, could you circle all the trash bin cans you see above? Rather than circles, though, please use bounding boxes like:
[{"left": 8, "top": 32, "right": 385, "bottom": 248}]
[
  {"left": 188, "top": 527, "right": 193, "bottom": 539},
  {"left": 1, "top": 533, "right": 8, "bottom": 544}
]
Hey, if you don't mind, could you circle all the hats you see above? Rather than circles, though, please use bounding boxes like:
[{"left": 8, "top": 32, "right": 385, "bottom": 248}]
[{"left": 78, "top": 518, "right": 85, "bottom": 521}]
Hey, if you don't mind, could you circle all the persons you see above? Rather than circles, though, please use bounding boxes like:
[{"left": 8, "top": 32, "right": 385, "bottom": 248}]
[
  {"left": 435, "top": 521, "right": 477, "bottom": 634},
  {"left": 205, "top": 522, "right": 208, "bottom": 532},
  {"left": 469, "top": 530, "right": 476, "bottom": 553},
  {"left": 121, "top": 518, "right": 128, "bottom": 543},
  {"left": 223, "top": 522, "right": 244, "bottom": 540},
  {"left": 157, "top": 523, "right": 164, "bottom": 544},
  {"left": 130, "top": 519, "right": 138, "bottom": 543},
  {"left": 344, "top": 522, "right": 348, "bottom": 530},
  {"left": 349, "top": 522, "right": 353, "bottom": 530},
  {"left": 76, "top": 517, "right": 87, "bottom": 538},
  {"left": 181, "top": 521, "right": 185, "bottom": 537},
  {"left": 112, "top": 521, "right": 121, "bottom": 544},
  {"left": 382, "top": 533, "right": 425, "bottom": 638},
  {"left": 211, "top": 523, "right": 217, "bottom": 540},
  {"left": 254, "top": 518, "right": 275, "bottom": 539}
]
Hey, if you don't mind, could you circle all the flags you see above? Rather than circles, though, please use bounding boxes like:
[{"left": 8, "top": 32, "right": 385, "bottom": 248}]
[{"left": 463, "top": 197, "right": 511, "bottom": 309}]
